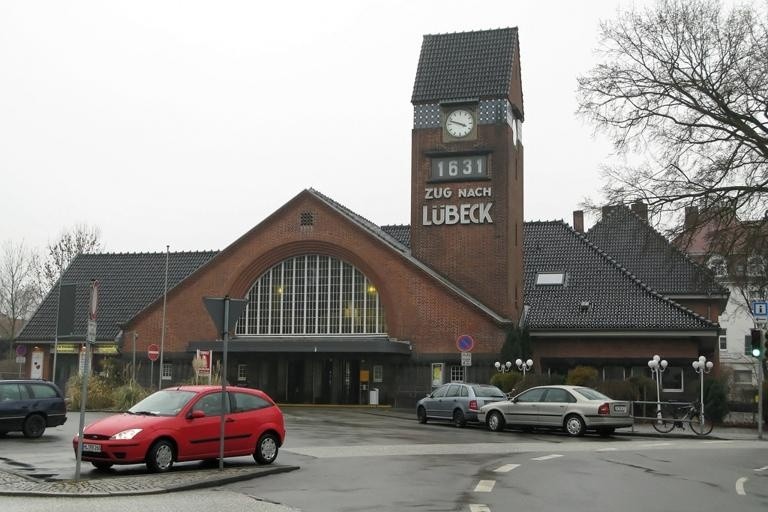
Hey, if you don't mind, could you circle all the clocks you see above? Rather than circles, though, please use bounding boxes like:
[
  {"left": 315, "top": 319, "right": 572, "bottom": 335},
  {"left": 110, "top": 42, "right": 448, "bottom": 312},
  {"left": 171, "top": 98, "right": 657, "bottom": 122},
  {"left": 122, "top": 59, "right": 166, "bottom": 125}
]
[{"left": 445, "top": 109, "right": 475, "bottom": 138}]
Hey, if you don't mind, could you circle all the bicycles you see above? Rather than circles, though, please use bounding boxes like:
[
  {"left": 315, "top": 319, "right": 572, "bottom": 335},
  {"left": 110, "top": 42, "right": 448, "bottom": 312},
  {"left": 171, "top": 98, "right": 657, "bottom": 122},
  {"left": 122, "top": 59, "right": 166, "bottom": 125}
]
[{"left": 650, "top": 398, "right": 715, "bottom": 436}]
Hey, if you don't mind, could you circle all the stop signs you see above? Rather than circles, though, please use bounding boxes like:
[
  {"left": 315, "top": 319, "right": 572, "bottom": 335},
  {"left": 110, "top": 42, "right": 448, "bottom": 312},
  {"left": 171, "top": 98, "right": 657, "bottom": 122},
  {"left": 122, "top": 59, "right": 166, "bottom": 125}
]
[{"left": 146, "top": 343, "right": 161, "bottom": 361}]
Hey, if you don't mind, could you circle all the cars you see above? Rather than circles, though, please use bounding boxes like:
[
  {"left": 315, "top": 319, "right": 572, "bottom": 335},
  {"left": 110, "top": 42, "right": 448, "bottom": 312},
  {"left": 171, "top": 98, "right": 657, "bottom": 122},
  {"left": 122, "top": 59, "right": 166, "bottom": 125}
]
[
  {"left": 71, "top": 382, "right": 286, "bottom": 473},
  {"left": 476, "top": 382, "right": 636, "bottom": 438}
]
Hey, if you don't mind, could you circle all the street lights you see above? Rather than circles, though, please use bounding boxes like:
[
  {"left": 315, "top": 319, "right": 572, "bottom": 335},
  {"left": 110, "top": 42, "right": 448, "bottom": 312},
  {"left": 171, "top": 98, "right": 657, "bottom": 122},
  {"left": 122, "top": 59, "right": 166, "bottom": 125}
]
[
  {"left": 646, "top": 353, "right": 670, "bottom": 426},
  {"left": 514, "top": 357, "right": 534, "bottom": 382},
  {"left": 493, "top": 359, "right": 512, "bottom": 374},
  {"left": 690, "top": 354, "right": 715, "bottom": 427}
]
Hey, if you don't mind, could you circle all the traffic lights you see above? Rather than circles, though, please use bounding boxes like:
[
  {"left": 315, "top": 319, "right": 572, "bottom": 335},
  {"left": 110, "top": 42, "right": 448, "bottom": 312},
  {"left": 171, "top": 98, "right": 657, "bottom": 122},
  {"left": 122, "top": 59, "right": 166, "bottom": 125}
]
[
  {"left": 760, "top": 329, "right": 768, "bottom": 359},
  {"left": 751, "top": 328, "right": 763, "bottom": 359}
]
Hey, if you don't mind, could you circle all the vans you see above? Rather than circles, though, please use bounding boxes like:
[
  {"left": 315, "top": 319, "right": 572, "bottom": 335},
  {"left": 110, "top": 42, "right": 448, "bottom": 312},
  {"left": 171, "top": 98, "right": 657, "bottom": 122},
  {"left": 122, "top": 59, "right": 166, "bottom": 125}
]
[
  {"left": 415, "top": 380, "right": 509, "bottom": 429},
  {"left": 0, "top": 377, "right": 67, "bottom": 439}
]
